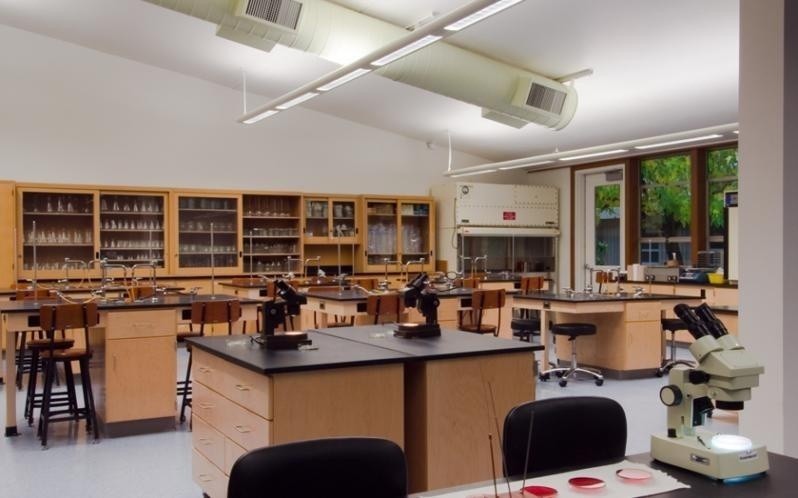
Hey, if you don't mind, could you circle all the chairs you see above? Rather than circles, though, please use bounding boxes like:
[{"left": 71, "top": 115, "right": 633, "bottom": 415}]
[
  {"left": 454, "top": 279, "right": 506, "bottom": 336},
  {"left": 521, "top": 277, "right": 543, "bottom": 292},
  {"left": 228, "top": 439, "right": 407, "bottom": 498},
  {"left": 177, "top": 300, "right": 244, "bottom": 431},
  {"left": 503, "top": 397, "right": 627, "bottom": 477},
  {"left": 11, "top": 282, "right": 100, "bottom": 448}
]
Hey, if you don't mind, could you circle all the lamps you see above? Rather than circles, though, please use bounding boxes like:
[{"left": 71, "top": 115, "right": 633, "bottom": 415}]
[
  {"left": 238, "top": 1, "right": 523, "bottom": 126},
  {"left": 444, "top": 121, "right": 738, "bottom": 178}
]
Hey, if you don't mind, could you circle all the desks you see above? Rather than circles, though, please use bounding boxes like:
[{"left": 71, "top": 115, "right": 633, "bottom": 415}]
[
  {"left": 0, "top": 291, "right": 264, "bottom": 436},
  {"left": 513, "top": 294, "right": 706, "bottom": 378},
  {"left": 183, "top": 322, "right": 545, "bottom": 498},
  {"left": 409, "top": 451, "right": 797, "bottom": 497}
]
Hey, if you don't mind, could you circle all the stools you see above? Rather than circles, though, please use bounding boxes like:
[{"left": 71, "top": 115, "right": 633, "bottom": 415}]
[
  {"left": 512, "top": 319, "right": 605, "bottom": 387},
  {"left": 656, "top": 319, "right": 697, "bottom": 379}
]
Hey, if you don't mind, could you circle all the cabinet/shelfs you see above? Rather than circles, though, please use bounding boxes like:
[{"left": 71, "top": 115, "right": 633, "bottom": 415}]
[
  {"left": 1, "top": 180, "right": 438, "bottom": 338},
  {"left": 599, "top": 281, "right": 739, "bottom": 312}
]
